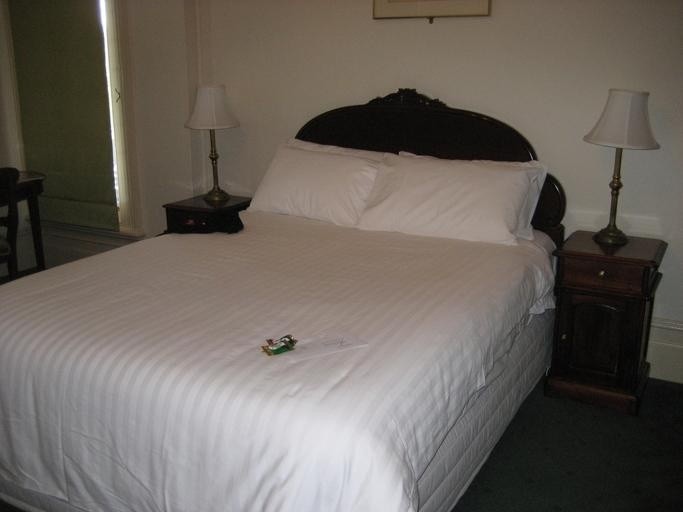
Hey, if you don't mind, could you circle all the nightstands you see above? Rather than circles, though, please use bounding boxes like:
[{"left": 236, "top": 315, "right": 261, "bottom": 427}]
[
  {"left": 162, "top": 192, "right": 253, "bottom": 233},
  {"left": 544, "top": 231, "right": 669, "bottom": 416}
]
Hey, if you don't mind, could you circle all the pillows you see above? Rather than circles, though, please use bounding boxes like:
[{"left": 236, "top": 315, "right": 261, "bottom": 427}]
[
  {"left": 416, "top": 155, "right": 546, "bottom": 241},
  {"left": 288, "top": 136, "right": 398, "bottom": 206},
  {"left": 248, "top": 145, "right": 378, "bottom": 228},
  {"left": 359, "top": 161, "right": 540, "bottom": 248}
]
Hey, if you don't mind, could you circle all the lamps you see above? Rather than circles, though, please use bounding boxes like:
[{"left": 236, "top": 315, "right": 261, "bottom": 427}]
[
  {"left": 583, "top": 87, "right": 661, "bottom": 247},
  {"left": 184, "top": 83, "right": 244, "bottom": 204}
]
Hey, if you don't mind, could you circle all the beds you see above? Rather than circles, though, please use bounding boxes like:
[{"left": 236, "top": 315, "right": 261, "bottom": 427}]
[{"left": 0, "top": 89, "right": 565, "bottom": 511}]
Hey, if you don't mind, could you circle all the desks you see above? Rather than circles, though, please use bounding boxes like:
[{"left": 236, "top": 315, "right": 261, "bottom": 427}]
[{"left": 0, "top": 171, "right": 45, "bottom": 282}]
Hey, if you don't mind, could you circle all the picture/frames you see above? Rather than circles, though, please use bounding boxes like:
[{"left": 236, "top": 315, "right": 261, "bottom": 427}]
[{"left": 374, "top": 0, "right": 491, "bottom": 25}]
